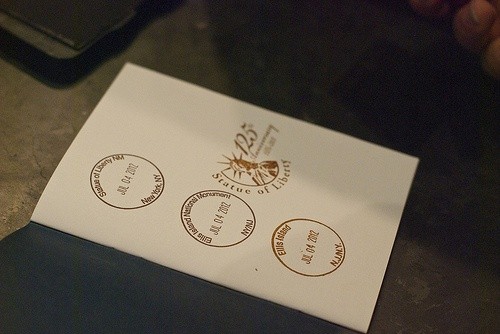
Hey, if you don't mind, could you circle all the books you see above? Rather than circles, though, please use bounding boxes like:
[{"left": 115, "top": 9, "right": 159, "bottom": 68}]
[
  {"left": 0, "top": 60, "right": 420, "bottom": 334},
  {"left": 1, "top": 0, "right": 178, "bottom": 88}
]
